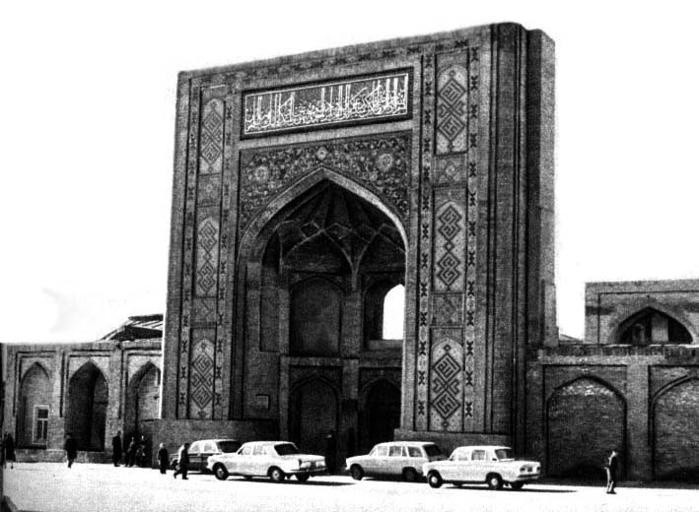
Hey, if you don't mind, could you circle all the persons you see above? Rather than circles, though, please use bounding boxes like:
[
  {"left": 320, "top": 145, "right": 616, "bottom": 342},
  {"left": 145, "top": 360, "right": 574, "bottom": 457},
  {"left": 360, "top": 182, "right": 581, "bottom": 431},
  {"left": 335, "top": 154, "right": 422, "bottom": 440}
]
[
  {"left": 136, "top": 433, "right": 148, "bottom": 468},
  {"left": 173, "top": 442, "right": 189, "bottom": 479},
  {"left": 125, "top": 436, "right": 137, "bottom": 467},
  {"left": 602, "top": 448, "right": 619, "bottom": 494},
  {"left": 157, "top": 442, "right": 170, "bottom": 474},
  {"left": 111, "top": 430, "right": 122, "bottom": 466},
  {"left": 63, "top": 433, "right": 77, "bottom": 467},
  {"left": 3, "top": 432, "right": 16, "bottom": 469}
]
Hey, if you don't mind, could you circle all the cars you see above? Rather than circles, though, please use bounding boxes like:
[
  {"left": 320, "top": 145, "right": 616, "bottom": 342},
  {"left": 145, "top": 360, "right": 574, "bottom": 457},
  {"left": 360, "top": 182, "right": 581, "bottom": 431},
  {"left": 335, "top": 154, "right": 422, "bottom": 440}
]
[
  {"left": 169, "top": 438, "right": 242, "bottom": 475},
  {"left": 415, "top": 446, "right": 543, "bottom": 490},
  {"left": 345, "top": 440, "right": 448, "bottom": 482}
]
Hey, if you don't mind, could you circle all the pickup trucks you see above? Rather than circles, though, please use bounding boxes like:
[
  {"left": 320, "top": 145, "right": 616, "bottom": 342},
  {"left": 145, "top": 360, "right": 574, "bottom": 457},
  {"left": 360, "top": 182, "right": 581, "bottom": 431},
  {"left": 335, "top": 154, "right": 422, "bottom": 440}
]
[{"left": 205, "top": 441, "right": 328, "bottom": 483}]
